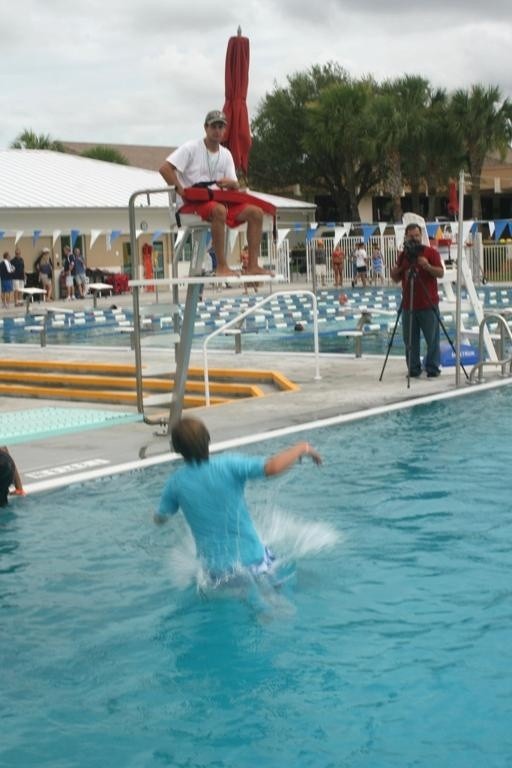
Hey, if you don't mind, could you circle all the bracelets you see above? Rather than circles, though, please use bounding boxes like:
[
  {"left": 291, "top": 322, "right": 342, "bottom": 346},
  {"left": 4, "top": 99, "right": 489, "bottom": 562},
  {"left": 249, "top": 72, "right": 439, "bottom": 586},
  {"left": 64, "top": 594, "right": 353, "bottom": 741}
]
[{"left": 422, "top": 264, "right": 431, "bottom": 271}]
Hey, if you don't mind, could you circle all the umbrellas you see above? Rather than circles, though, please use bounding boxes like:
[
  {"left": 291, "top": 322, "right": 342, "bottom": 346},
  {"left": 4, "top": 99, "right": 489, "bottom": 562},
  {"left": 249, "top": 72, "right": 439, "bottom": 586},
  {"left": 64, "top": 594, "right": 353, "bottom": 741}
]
[
  {"left": 214, "top": 23, "right": 254, "bottom": 190},
  {"left": 447, "top": 181, "right": 460, "bottom": 224}
]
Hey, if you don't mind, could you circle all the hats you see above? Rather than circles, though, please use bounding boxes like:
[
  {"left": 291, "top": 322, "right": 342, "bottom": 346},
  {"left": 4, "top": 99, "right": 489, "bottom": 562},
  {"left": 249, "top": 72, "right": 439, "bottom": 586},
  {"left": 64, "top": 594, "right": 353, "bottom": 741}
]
[{"left": 205, "top": 111, "right": 228, "bottom": 126}]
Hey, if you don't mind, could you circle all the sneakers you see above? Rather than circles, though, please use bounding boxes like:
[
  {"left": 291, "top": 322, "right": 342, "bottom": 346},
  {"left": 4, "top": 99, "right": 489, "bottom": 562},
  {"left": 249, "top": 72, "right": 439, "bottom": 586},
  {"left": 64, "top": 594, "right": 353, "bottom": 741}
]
[
  {"left": 3, "top": 295, "right": 86, "bottom": 309},
  {"left": 406, "top": 369, "right": 423, "bottom": 379},
  {"left": 426, "top": 370, "right": 442, "bottom": 381},
  {"left": 335, "top": 279, "right": 378, "bottom": 288}
]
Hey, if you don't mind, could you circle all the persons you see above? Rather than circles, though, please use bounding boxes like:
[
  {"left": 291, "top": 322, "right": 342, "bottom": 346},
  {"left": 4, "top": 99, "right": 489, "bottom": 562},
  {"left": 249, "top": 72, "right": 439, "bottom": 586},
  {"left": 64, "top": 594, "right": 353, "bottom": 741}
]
[
  {"left": 330, "top": 241, "right": 345, "bottom": 286},
  {"left": 371, "top": 245, "right": 386, "bottom": 285},
  {"left": 1, "top": 252, "right": 17, "bottom": 309},
  {"left": 59, "top": 244, "right": 78, "bottom": 301},
  {"left": 314, "top": 239, "right": 327, "bottom": 287},
  {"left": 389, "top": 223, "right": 445, "bottom": 380},
  {"left": 70, "top": 246, "right": 88, "bottom": 299},
  {"left": 151, "top": 416, "right": 325, "bottom": 582},
  {"left": 8, "top": 246, "right": 26, "bottom": 308},
  {"left": 236, "top": 246, "right": 258, "bottom": 295},
  {"left": 349, "top": 241, "right": 370, "bottom": 288},
  {"left": 349, "top": 243, "right": 360, "bottom": 277},
  {"left": 33, "top": 247, "right": 55, "bottom": 302},
  {"left": 368, "top": 243, "right": 378, "bottom": 262},
  {"left": 156, "top": 110, "right": 278, "bottom": 278}
]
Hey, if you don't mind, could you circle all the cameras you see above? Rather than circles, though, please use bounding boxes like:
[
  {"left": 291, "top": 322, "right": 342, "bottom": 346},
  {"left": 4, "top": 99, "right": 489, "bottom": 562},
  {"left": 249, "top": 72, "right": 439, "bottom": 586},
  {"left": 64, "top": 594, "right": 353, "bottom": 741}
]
[{"left": 402, "top": 239, "right": 424, "bottom": 263}]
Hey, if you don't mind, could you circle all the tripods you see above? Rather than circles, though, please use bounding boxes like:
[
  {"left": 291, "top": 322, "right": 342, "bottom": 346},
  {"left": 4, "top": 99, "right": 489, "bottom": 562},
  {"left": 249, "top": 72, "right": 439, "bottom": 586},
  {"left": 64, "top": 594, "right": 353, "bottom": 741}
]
[{"left": 376, "top": 266, "right": 470, "bottom": 391}]
